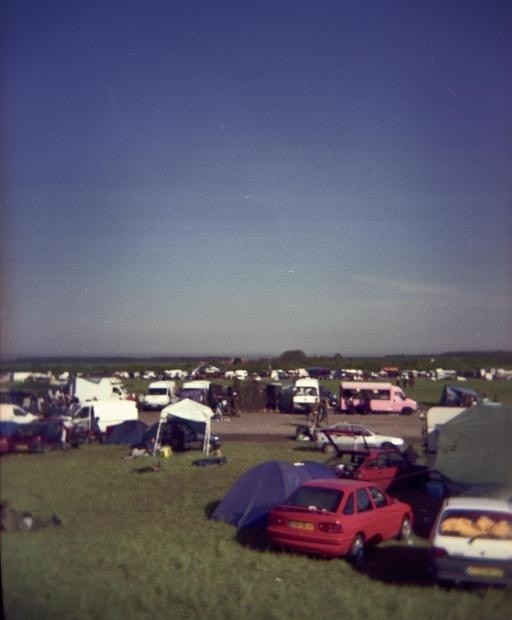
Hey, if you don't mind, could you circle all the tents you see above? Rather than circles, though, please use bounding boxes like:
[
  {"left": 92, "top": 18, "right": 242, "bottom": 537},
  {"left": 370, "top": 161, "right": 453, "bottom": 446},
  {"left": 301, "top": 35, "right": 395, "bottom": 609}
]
[
  {"left": 440, "top": 384, "right": 481, "bottom": 408},
  {"left": 104, "top": 419, "right": 149, "bottom": 447},
  {"left": 210, "top": 459, "right": 340, "bottom": 530}
]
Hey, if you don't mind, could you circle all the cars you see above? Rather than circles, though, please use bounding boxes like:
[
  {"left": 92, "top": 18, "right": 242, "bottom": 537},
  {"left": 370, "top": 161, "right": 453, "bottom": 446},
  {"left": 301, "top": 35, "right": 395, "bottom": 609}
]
[
  {"left": 316, "top": 422, "right": 405, "bottom": 454},
  {"left": 326, "top": 451, "right": 417, "bottom": 493},
  {"left": 426, "top": 496, "right": 512, "bottom": 590},
  {"left": 266, "top": 477, "right": 414, "bottom": 560}
]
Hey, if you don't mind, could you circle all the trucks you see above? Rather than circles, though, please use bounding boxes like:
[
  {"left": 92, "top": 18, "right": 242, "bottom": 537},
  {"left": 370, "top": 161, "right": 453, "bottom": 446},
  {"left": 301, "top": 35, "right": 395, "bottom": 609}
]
[
  {"left": 180, "top": 379, "right": 210, "bottom": 408},
  {"left": 269, "top": 368, "right": 458, "bottom": 380},
  {"left": 143, "top": 363, "right": 248, "bottom": 381},
  {"left": 143, "top": 380, "right": 175, "bottom": 410},
  {"left": 67, "top": 399, "right": 138, "bottom": 440},
  {"left": 70, "top": 375, "right": 129, "bottom": 400},
  {"left": 339, "top": 382, "right": 417, "bottom": 414},
  {"left": 292, "top": 378, "right": 320, "bottom": 412}
]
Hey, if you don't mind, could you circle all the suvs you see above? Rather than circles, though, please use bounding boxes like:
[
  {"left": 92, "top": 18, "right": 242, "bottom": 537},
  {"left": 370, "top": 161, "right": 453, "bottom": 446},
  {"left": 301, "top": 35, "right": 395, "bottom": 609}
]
[{"left": 0, "top": 389, "right": 70, "bottom": 453}]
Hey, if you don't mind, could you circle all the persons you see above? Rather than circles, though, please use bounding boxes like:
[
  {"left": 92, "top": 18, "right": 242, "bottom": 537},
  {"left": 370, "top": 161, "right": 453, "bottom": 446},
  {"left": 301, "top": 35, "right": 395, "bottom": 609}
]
[
  {"left": 146, "top": 436, "right": 156, "bottom": 453},
  {"left": 459, "top": 393, "right": 478, "bottom": 407},
  {"left": 171, "top": 425, "right": 186, "bottom": 452}
]
